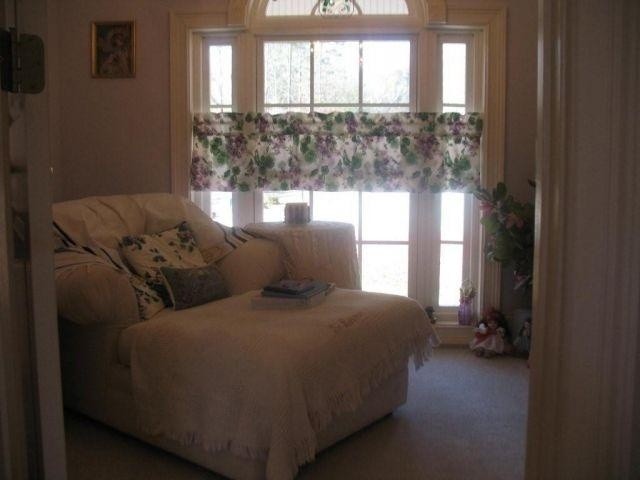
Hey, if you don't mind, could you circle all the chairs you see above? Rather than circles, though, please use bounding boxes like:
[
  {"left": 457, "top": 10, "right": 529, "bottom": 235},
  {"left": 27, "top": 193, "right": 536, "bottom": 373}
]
[{"left": 53, "top": 193, "right": 422, "bottom": 480}]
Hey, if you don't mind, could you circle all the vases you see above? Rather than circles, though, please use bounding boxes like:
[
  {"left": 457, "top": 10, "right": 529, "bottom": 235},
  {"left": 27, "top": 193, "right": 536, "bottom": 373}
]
[{"left": 514, "top": 308, "right": 531, "bottom": 335}]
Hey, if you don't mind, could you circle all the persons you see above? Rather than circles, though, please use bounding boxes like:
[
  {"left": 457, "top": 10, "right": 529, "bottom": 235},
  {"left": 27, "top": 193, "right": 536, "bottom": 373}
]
[
  {"left": 513, "top": 318, "right": 530, "bottom": 357},
  {"left": 470, "top": 313, "right": 506, "bottom": 357}
]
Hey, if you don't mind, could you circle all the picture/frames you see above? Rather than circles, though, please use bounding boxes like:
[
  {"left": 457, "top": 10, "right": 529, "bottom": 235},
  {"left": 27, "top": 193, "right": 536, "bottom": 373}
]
[{"left": 89, "top": 19, "right": 136, "bottom": 78}]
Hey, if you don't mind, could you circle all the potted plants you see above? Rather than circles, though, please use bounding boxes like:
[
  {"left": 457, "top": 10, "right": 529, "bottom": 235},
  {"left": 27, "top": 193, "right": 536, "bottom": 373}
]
[{"left": 458, "top": 279, "right": 476, "bottom": 323}]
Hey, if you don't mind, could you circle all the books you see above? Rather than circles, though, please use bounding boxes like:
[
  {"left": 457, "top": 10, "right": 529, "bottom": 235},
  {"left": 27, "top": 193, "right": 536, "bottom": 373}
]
[{"left": 251, "top": 279, "right": 334, "bottom": 310}]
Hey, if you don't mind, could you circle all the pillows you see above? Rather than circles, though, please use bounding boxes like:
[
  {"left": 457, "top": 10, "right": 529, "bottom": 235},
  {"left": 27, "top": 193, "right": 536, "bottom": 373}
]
[{"left": 120, "top": 226, "right": 233, "bottom": 318}]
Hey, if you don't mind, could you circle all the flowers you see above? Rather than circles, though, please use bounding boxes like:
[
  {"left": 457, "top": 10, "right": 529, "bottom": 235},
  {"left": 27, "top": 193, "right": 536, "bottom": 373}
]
[{"left": 473, "top": 176, "right": 535, "bottom": 295}]
